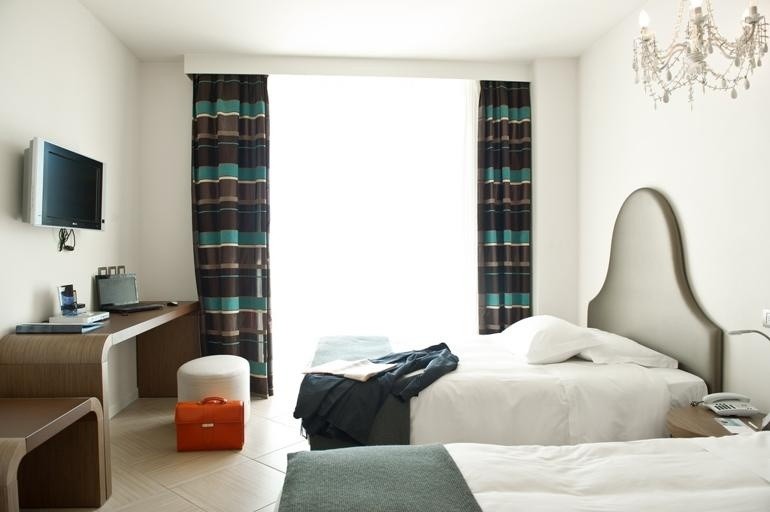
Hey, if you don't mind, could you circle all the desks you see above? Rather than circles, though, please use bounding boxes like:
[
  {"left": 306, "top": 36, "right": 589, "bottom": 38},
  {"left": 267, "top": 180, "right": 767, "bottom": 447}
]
[
  {"left": 0, "top": 300, "right": 203, "bottom": 500},
  {"left": 0, "top": 397, "right": 106, "bottom": 512}
]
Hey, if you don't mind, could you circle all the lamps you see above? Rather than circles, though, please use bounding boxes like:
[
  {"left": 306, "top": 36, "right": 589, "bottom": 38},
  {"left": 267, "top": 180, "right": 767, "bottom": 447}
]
[{"left": 631, "top": 0, "right": 770, "bottom": 110}]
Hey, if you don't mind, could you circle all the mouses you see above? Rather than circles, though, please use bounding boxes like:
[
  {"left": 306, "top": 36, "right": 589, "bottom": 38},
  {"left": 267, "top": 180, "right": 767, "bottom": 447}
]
[{"left": 167, "top": 301, "right": 179, "bottom": 305}]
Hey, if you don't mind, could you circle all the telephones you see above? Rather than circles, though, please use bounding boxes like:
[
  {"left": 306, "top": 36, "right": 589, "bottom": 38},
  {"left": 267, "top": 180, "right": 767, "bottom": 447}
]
[{"left": 703, "top": 392, "right": 758, "bottom": 416}]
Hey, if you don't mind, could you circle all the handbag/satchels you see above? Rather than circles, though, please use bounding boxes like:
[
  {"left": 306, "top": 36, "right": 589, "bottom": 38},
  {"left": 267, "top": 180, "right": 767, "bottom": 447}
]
[{"left": 175, "top": 397, "right": 244, "bottom": 451}]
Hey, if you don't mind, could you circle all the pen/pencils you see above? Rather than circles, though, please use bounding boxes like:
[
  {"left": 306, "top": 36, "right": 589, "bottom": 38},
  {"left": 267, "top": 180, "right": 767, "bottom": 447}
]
[{"left": 748, "top": 421, "right": 758, "bottom": 429}]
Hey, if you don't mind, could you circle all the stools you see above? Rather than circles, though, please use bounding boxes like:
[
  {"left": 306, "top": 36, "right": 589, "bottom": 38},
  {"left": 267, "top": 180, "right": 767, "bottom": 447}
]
[{"left": 177, "top": 355, "right": 250, "bottom": 426}]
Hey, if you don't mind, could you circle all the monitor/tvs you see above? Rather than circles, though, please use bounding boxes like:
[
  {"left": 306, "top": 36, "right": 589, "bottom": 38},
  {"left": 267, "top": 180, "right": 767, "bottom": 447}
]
[{"left": 20, "top": 137, "right": 107, "bottom": 232}]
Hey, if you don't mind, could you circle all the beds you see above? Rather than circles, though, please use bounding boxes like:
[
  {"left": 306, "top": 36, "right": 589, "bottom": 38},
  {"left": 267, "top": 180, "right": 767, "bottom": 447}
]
[
  {"left": 294, "top": 187, "right": 723, "bottom": 452},
  {"left": 273, "top": 430, "right": 770, "bottom": 512}
]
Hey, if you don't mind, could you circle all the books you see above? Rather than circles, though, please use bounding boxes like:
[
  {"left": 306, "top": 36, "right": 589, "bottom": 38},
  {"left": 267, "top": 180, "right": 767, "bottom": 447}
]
[{"left": 302, "top": 359, "right": 397, "bottom": 382}]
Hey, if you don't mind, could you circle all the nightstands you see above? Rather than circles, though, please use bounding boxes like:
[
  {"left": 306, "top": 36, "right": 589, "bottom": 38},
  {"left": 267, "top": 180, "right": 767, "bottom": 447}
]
[{"left": 664, "top": 406, "right": 767, "bottom": 438}]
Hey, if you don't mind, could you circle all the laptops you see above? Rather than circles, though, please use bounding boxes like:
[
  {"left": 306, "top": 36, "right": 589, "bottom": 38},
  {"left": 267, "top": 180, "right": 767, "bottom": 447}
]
[{"left": 95, "top": 273, "right": 164, "bottom": 313}]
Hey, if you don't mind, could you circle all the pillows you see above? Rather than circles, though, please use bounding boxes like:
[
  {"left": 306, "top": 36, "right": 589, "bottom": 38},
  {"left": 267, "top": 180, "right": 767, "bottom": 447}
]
[{"left": 496, "top": 315, "right": 679, "bottom": 370}]
